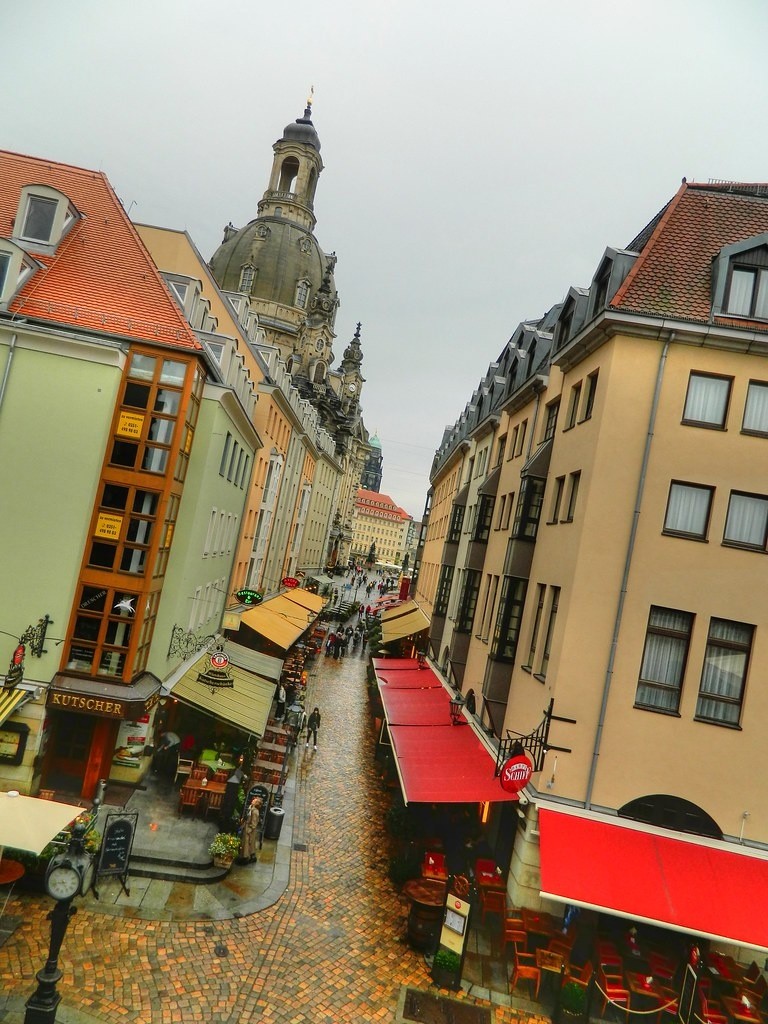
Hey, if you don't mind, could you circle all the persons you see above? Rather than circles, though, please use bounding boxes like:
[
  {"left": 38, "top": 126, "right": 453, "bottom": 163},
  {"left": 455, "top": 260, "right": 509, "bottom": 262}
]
[
  {"left": 293, "top": 706, "right": 321, "bottom": 750},
  {"left": 324, "top": 603, "right": 372, "bottom": 660},
  {"left": 402, "top": 552, "right": 409, "bottom": 571},
  {"left": 351, "top": 565, "right": 394, "bottom": 598},
  {"left": 327, "top": 570, "right": 339, "bottom": 606},
  {"left": 275, "top": 681, "right": 297, "bottom": 718},
  {"left": 239, "top": 797, "right": 263, "bottom": 866},
  {"left": 158, "top": 725, "right": 181, "bottom": 770}
]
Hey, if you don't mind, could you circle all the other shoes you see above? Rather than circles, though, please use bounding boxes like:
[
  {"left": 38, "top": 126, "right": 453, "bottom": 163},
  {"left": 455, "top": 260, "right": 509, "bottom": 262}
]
[
  {"left": 306, "top": 743, "right": 309, "bottom": 747},
  {"left": 313, "top": 745, "right": 318, "bottom": 750}
]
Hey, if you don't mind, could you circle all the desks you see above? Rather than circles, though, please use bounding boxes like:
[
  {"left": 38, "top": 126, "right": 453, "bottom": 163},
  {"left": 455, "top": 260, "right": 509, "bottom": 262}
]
[
  {"left": 283, "top": 644, "right": 315, "bottom": 684},
  {"left": 415, "top": 834, "right": 763, "bottom": 1024},
  {"left": 182, "top": 764, "right": 233, "bottom": 794},
  {"left": 249, "top": 725, "right": 291, "bottom": 795},
  {"left": 0, "top": 858, "right": 25, "bottom": 918}
]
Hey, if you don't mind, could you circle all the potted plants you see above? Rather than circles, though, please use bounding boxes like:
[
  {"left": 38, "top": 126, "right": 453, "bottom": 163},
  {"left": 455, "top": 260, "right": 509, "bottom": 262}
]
[{"left": 209, "top": 833, "right": 242, "bottom": 870}]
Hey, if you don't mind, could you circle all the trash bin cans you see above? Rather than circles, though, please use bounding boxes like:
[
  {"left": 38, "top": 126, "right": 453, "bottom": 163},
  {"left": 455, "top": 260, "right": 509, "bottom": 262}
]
[
  {"left": 95, "top": 779, "right": 107, "bottom": 805},
  {"left": 264, "top": 807, "right": 285, "bottom": 840}
]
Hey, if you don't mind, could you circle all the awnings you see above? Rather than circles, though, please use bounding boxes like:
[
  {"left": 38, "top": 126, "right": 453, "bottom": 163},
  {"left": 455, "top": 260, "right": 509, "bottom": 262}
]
[
  {"left": 0, "top": 791, "right": 87, "bottom": 858},
  {"left": 312, "top": 575, "right": 335, "bottom": 584},
  {"left": 241, "top": 587, "right": 330, "bottom": 653},
  {"left": 381, "top": 600, "right": 432, "bottom": 645},
  {"left": 159, "top": 633, "right": 285, "bottom": 740},
  {"left": 535, "top": 798, "right": 768, "bottom": 954},
  {"left": 0, "top": 682, "right": 38, "bottom": 727},
  {"left": 372, "top": 656, "right": 528, "bottom": 808}
]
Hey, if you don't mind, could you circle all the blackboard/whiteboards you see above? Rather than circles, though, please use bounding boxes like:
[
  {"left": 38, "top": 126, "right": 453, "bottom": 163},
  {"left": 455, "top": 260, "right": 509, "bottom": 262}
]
[
  {"left": 98, "top": 817, "right": 133, "bottom": 876},
  {"left": 241, "top": 784, "right": 270, "bottom": 832}
]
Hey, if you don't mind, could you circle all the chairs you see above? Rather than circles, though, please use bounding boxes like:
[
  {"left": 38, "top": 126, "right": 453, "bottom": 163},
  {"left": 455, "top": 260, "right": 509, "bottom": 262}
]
[
  {"left": 174, "top": 728, "right": 246, "bottom": 823},
  {"left": 249, "top": 718, "right": 295, "bottom": 829},
  {"left": 419, "top": 852, "right": 768, "bottom": 1024}
]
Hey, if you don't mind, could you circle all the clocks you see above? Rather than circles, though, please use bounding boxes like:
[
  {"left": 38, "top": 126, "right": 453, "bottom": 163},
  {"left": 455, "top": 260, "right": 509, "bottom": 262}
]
[{"left": 44, "top": 852, "right": 96, "bottom": 901}]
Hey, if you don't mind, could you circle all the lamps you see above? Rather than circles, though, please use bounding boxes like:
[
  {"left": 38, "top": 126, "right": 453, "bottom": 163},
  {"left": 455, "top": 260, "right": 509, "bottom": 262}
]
[
  {"left": 449, "top": 693, "right": 472, "bottom": 727},
  {"left": 417, "top": 648, "right": 429, "bottom": 671}
]
